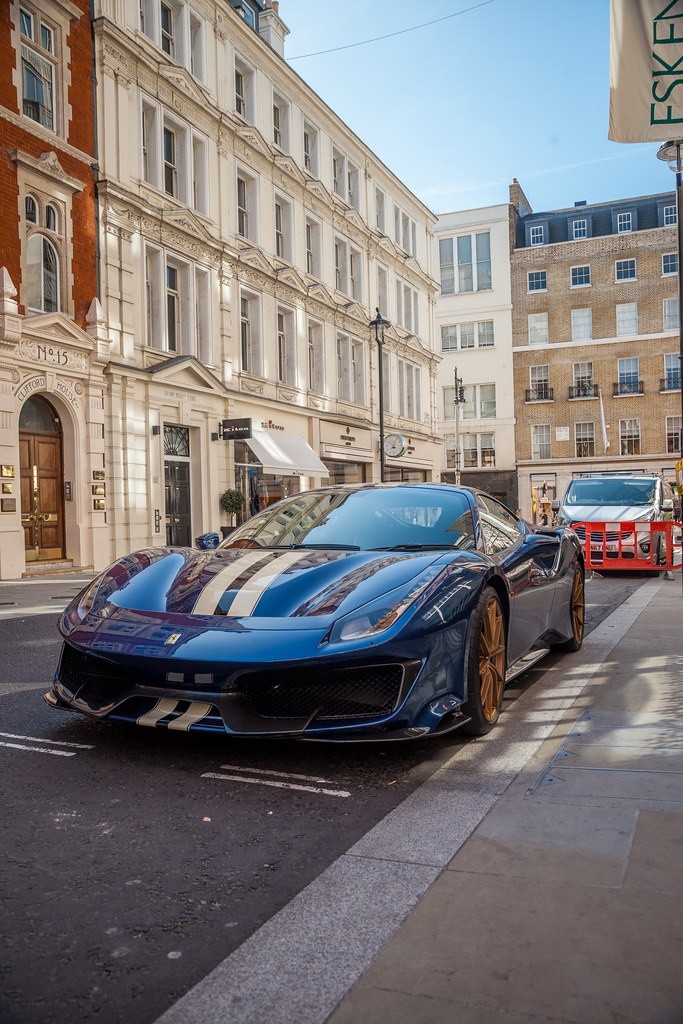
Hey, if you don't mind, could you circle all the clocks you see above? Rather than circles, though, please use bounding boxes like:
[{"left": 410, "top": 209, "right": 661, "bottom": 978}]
[{"left": 385, "top": 434, "right": 407, "bottom": 457}]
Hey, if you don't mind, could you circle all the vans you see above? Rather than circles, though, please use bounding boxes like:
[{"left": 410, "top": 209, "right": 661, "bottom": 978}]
[{"left": 550, "top": 470, "right": 676, "bottom": 579}]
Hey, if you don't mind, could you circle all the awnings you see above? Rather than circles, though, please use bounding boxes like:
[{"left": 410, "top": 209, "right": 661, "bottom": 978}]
[{"left": 243, "top": 429, "right": 330, "bottom": 478}]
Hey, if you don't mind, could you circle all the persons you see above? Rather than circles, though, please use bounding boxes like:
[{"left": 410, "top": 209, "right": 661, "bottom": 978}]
[
  {"left": 538, "top": 508, "right": 548, "bottom": 528},
  {"left": 673, "top": 499, "right": 681, "bottom": 523},
  {"left": 516, "top": 509, "right": 522, "bottom": 518}
]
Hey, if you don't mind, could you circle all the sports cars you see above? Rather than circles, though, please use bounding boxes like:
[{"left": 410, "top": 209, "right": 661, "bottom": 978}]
[{"left": 46, "top": 482, "right": 589, "bottom": 749}]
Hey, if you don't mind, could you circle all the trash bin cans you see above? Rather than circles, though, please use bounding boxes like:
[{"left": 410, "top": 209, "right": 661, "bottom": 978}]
[{"left": 196, "top": 533, "right": 220, "bottom": 550}]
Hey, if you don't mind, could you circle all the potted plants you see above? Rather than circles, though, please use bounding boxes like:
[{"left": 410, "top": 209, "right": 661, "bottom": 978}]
[{"left": 219, "top": 488, "right": 246, "bottom": 539}]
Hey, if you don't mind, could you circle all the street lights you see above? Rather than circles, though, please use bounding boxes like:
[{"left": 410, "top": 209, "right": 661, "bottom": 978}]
[
  {"left": 453, "top": 367, "right": 466, "bottom": 485},
  {"left": 367, "top": 310, "right": 387, "bottom": 484}
]
